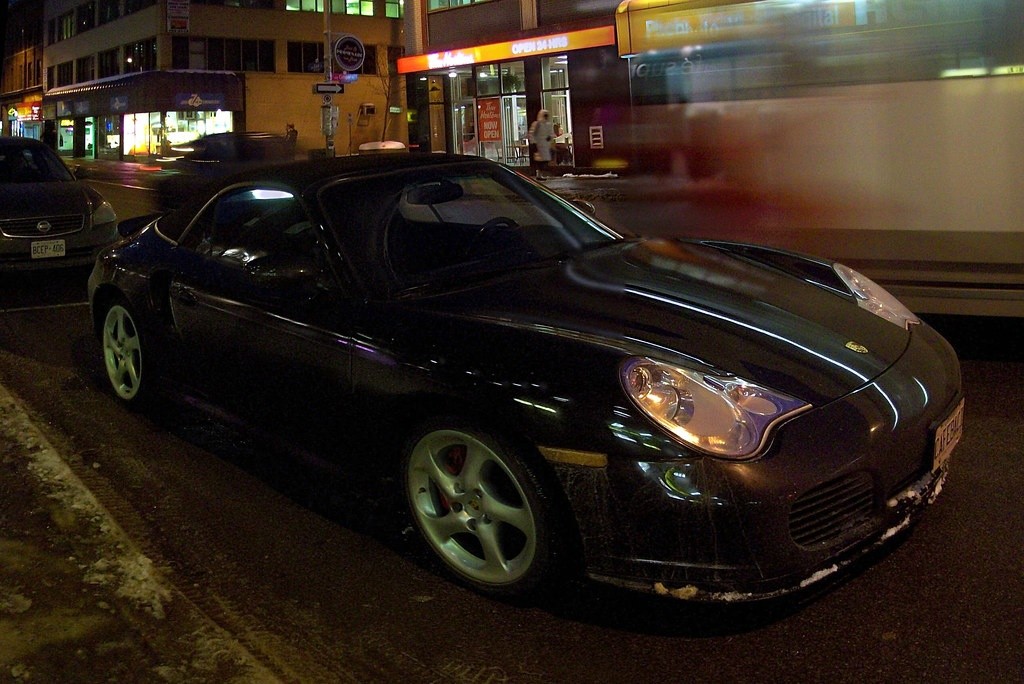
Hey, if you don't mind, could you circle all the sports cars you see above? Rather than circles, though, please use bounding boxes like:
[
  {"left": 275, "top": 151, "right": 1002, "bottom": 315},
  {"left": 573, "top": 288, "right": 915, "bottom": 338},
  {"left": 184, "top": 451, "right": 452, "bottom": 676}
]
[{"left": 84, "top": 149, "right": 969, "bottom": 625}]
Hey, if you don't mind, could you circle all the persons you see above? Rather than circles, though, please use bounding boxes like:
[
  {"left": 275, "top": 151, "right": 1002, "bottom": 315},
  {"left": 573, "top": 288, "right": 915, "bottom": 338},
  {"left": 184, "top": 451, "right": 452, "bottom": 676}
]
[
  {"left": 527, "top": 109, "right": 557, "bottom": 183},
  {"left": 282, "top": 122, "right": 299, "bottom": 160}
]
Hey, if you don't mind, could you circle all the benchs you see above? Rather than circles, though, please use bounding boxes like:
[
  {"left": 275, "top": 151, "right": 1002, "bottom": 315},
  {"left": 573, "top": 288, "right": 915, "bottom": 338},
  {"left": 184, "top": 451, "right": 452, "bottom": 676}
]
[{"left": 496, "top": 141, "right": 527, "bottom": 166}]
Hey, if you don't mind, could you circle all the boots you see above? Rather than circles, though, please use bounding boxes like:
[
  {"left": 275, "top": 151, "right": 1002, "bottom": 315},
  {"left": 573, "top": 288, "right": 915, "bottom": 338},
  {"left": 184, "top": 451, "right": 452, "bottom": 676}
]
[{"left": 536, "top": 170, "right": 547, "bottom": 180}]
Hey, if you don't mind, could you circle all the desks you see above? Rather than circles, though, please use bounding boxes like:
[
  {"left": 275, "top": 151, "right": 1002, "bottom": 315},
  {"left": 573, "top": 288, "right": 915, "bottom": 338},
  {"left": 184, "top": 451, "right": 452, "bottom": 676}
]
[{"left": 507, "top": 145, "right": 529, "bottom": 165}]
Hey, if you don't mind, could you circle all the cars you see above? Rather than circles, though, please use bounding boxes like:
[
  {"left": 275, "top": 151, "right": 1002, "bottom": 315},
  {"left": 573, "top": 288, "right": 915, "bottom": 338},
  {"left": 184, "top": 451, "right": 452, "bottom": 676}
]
[{"left": 0, "top": 135, "right": 119, "bottom": 283}]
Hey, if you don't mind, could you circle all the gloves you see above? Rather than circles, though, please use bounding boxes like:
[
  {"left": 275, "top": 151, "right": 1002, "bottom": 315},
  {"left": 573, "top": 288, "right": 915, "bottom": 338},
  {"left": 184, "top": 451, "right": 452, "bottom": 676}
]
[
  {"left": 546, "top": 136, "right": 551, "bottom": 141},
  {"left": 530, "top": 143, "right": 538, "bottom": 153}
]
